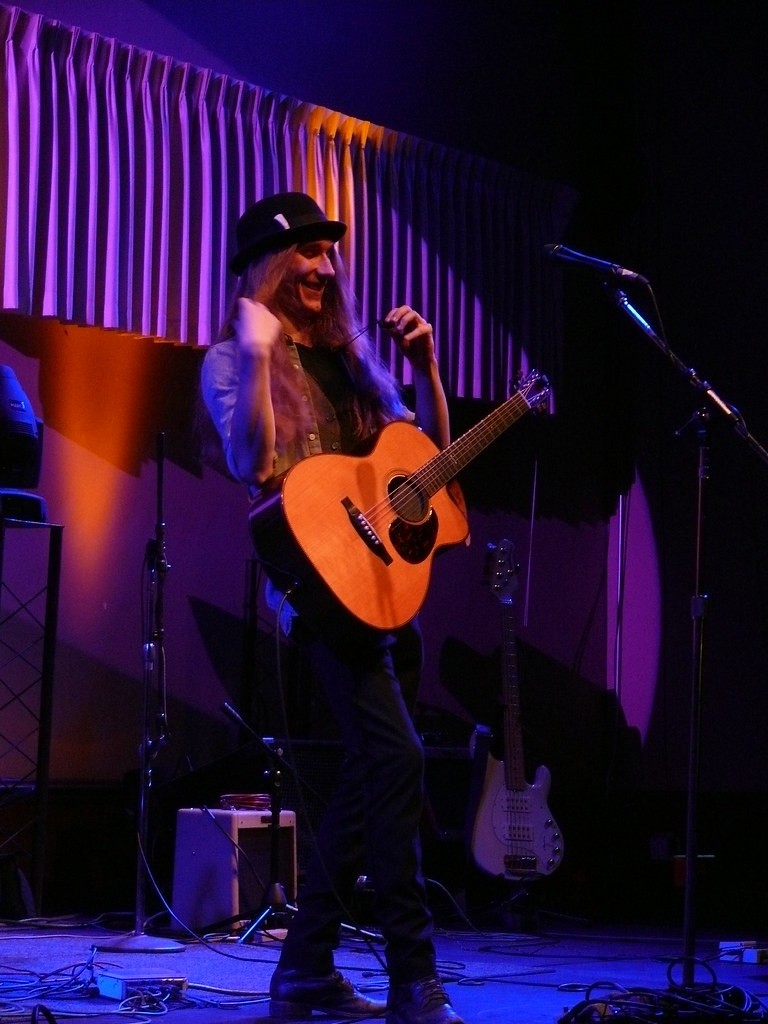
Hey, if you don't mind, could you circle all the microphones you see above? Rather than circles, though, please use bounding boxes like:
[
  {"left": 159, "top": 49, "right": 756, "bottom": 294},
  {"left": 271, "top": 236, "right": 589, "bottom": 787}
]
[
  {"left": 140, "top": 733, "right": 172, "bottom": 760},
  {"left": 356, "top": 874, "right": 376, "bottom": 891},
  {"left": 541, "top": 244, "right": 649, "bottom": 286}
]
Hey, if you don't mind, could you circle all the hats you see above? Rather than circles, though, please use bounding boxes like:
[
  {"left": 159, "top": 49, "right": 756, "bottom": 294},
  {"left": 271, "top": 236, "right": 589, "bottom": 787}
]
[{"left": 228, "top": 190, "right": 347, "bottom": 275}]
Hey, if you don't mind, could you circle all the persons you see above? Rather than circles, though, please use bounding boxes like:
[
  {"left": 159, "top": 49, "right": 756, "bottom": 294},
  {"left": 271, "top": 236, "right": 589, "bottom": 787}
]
[{"left": 200, "top": 192, "right": 467, "bottom": 1024}]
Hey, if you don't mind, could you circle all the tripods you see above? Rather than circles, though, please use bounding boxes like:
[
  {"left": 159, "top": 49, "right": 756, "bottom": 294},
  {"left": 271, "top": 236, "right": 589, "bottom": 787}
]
[{"left": 179, "top": 699, "right": 386, "bottom": 946}]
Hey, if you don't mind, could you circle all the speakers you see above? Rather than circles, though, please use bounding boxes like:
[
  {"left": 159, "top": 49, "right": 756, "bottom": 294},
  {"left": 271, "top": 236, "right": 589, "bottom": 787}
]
[
  {"left": 171, "top": 807, "right": 297, "bottom": 939},
  {"left": 258, "top": 734, "right": 477, "bottom": 930}
]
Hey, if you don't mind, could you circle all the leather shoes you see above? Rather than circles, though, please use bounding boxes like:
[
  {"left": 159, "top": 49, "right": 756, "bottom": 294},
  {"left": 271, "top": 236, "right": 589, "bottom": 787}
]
[
  {"left": 269, "top": 971, "right": 386, "bottom": 1020},
  {"left": 386, "top": 974, "right": 466, "bottom": 1024}
]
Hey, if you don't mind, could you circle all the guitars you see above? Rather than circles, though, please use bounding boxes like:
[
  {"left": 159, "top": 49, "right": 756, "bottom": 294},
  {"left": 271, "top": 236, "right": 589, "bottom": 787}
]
[
  {"left": 470, "top": 536, "right": 567, "bottom": 880},
  {"left": 246, "top": 365, "right": 556, "bottom": 635}
]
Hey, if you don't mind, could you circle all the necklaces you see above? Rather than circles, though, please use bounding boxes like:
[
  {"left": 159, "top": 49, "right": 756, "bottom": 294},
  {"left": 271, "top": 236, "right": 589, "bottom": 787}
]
[{"left": 333, "top": 317, "right": 394, "bottom": 355}]
